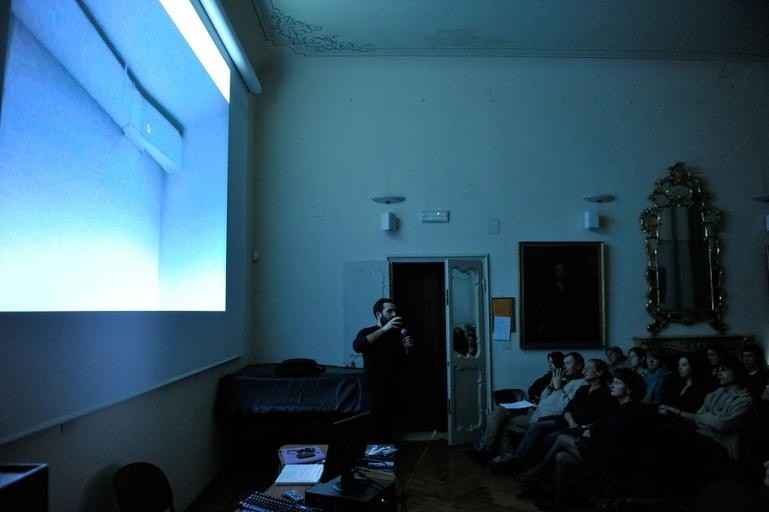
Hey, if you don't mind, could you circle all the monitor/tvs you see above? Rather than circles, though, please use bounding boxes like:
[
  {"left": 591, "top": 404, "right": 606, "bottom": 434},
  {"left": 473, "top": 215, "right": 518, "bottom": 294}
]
[{"left": 320, "top": 410, "right": 373, "bottom": 493}]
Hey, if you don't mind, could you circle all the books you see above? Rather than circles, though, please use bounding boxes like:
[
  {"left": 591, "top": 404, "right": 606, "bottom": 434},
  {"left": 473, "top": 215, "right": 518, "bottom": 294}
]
[{"left": 274, "top": 464, "right": 324, "bottom": 486}]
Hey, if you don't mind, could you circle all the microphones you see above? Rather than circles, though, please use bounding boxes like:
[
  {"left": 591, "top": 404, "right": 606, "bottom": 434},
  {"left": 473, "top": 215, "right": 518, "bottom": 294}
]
[{"left": 400, "top": 328, "right": 408, "bottom": 338}]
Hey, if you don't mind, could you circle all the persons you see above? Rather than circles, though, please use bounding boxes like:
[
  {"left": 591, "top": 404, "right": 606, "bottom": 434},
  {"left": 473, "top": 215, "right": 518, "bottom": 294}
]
[
  {"left": 353, "top": 298, "right": 415, "bottom": 426},
  {"left": 477, "top": 342, "right": 768, "bottom": 509}
]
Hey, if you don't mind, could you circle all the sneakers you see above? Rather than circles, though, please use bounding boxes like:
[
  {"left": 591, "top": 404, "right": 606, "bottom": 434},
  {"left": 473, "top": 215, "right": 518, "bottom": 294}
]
[{"left": 470, "top": 444, "right": 545, "bottom": 498}]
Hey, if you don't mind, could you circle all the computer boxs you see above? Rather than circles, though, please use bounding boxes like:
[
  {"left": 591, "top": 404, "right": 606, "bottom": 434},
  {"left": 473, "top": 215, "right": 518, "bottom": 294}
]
[{"left": 305, "top": 475, "right": 393, "bottom": 512}]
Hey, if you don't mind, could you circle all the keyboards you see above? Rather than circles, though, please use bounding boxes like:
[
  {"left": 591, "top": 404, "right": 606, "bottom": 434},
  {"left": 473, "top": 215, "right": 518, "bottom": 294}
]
[{"left": 238, "top": 491, "right": 324, "bottom": 512}]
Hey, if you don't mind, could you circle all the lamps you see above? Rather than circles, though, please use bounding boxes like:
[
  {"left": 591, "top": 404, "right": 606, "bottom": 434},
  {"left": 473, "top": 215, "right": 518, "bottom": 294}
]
[{"left": 583, "top": 208, "right": 601, "bottom": 232}]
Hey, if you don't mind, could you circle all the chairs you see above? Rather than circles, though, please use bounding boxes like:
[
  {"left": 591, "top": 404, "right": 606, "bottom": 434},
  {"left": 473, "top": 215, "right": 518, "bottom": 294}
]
[{"left": 493, "top": 388, "right": 529, "bottom": 409}]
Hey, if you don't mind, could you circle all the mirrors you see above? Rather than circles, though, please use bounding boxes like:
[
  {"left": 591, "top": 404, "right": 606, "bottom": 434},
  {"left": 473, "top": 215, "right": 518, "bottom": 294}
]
[{"left": 639, "top": 161, "right": 732, "bottom": 340}]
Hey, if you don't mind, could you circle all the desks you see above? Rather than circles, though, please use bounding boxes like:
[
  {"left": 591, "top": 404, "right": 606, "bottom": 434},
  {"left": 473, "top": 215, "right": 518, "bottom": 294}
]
[{"left": 187, "top": 466, "right": 390, "bottom": 512}]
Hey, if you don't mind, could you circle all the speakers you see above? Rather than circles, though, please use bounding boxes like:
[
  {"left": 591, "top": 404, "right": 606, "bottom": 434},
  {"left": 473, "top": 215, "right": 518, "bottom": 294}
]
[{"left": 584, "top": 210, "right": 600, "bottom": 230}]
